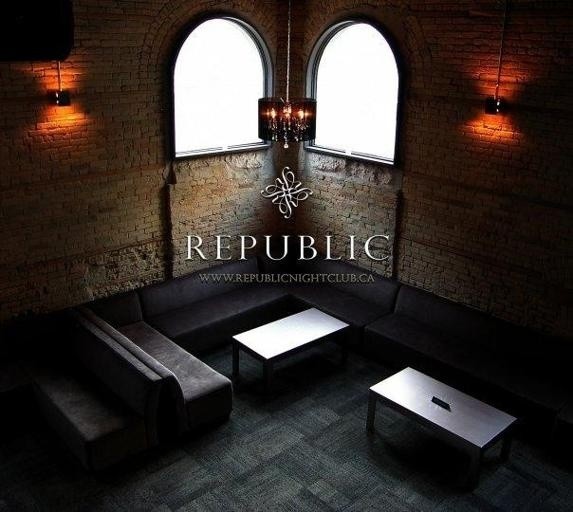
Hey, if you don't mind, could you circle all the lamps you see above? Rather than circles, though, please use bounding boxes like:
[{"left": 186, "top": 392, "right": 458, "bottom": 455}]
[
  {"left": 482, "top": 82, "right": 507, "bottom": 116},
  {"left": 254, "top": 18, "right": 318, "bottom": 147},
  {"left": 53, "top": 60, "right": 73, "bottom": 107}
]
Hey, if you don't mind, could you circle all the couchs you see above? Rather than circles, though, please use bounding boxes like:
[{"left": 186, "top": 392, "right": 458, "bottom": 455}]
[
  {"left": 137, "top": 253, "right": 288, "bottom": 353},
  {"left": 361, "top": 281, "right": 572, "bottom": 448},
  {"left": 78, "top": 288, "right": 233, "bottom": 440},
  {"left": 30, "top": 308, "right": 164, "bottom": 469},
  {"left": 283, "top": 245, "right": 400, "bottom": 355}
]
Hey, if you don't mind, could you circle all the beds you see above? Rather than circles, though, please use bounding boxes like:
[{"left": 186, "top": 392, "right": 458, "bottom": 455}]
[{"left": 361, "top": 362, "right": 521, "bottom": 490}]
